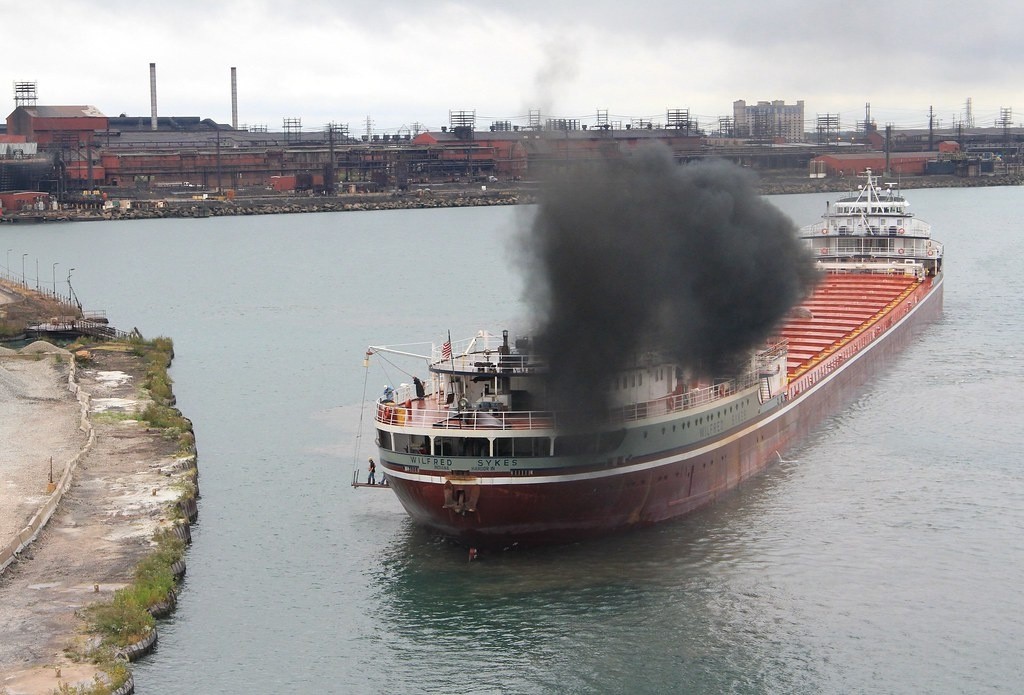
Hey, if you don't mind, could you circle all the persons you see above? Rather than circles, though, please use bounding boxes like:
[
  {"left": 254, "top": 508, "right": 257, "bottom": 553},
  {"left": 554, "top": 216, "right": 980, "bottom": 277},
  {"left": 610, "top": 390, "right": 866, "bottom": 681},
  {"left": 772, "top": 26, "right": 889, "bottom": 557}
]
[
  {"left": 383, "top": 384, "right": 395, "bottom": 401},
  {"left": 367, "top": 457, "right": 389, "bottom": 485},
  {"left": 412, "top": 376, "right": 425, "bottom": 401}
]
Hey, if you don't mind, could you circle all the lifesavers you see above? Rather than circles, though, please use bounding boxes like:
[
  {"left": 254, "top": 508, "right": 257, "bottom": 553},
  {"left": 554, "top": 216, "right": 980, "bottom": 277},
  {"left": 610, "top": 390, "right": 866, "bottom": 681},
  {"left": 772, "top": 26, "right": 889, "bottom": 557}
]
[
  {"left": 898, "top": 248, "right": 904, "bottom": 254},
  {"left": 482, "top": 349, "right": 492, "bottom": 358},
  {"left": 928, "top": 250, "right": 933, "bottom": 256},
  {"left": 822, "top": 248, "right": 828, "bottom": 254},
  {"left": 822, "top": 228, "right": 827, "bottom": 234},
  {"left": 899, "top": 228, "right": 905, "bottom": 234}
]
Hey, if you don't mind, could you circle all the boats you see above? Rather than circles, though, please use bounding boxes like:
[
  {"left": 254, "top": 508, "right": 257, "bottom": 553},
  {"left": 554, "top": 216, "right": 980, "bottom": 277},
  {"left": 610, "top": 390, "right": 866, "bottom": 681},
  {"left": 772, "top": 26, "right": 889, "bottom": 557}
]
[
  {"left": 24, "top": 314, "right": 116, "bottom": 340},
  {"left": 349, "top": 164, "right": 946, "bottom": 547}
]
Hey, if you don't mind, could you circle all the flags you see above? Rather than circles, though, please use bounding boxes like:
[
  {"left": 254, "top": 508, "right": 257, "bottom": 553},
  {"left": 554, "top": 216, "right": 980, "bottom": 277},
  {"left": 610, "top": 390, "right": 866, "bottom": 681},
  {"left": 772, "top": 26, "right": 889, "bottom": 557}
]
[{"left": 442, "top": 341, "right": 451, "bottom": 358}]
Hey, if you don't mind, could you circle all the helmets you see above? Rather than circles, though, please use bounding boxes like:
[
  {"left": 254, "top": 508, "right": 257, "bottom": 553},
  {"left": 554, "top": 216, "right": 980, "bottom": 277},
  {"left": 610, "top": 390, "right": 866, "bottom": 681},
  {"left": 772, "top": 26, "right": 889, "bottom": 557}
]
[
  {"left": 384, "top": 385, "right": 387, "bottom": 390},
  {"left": 369, "top": 457, "right": 372, "bottom": 460}
]
